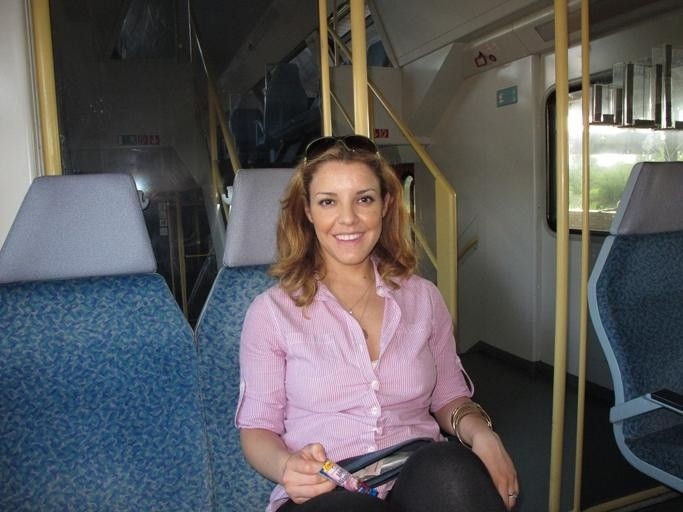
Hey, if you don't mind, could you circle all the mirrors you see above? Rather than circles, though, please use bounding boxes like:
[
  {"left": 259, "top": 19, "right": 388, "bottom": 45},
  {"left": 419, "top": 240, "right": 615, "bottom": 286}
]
[{"left": 31, "top": 2, "right": 330, "bottom": 330}]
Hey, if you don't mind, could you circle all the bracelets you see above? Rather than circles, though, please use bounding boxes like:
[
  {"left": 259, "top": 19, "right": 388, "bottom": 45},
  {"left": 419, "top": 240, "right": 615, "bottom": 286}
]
[{"left": 451, "top": 402, "right": 493, "bottom": 450}]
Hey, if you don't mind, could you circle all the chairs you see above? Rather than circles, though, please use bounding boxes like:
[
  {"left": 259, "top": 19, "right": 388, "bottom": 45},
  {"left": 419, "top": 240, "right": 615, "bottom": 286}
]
[
  {"left": 193, "top": 167, "right": 309, "bottom": 508},
  {"left": 1, "top": 172, "right": 207, "bottom": 511},
  {"left": 227, "top": 61, "right": 313, "bottom": 168},
  {"left": 586, "top": 159, "right": 682, "bottom": 491}
]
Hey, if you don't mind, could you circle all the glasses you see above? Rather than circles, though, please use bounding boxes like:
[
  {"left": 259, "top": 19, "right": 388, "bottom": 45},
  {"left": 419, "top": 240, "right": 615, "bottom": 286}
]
[{"left": 304, "top": 136, "right": 380, "bottom": 165}]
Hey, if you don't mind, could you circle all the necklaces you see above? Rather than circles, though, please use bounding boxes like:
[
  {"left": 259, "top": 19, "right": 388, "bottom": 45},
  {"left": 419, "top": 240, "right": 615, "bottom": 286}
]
[{"left": 329, "top": 281, "right": 375, "bottom": 339}]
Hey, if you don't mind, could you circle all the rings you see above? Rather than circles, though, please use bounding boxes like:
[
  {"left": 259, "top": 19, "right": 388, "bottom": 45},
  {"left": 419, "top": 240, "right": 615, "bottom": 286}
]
[{"left": 508, "top": 492, "right": 518, "bottom": 498}]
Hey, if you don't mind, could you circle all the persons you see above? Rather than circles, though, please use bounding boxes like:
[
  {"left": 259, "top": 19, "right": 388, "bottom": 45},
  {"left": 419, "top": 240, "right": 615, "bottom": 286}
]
[{"left": 235, "top": 136, "right": 521, "bottom": 512}]
